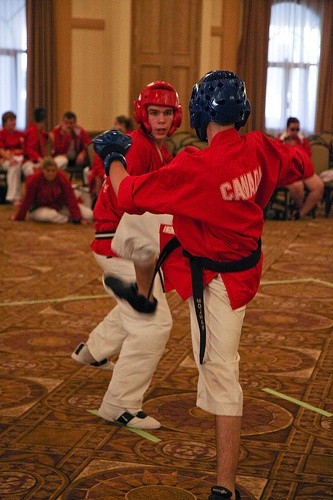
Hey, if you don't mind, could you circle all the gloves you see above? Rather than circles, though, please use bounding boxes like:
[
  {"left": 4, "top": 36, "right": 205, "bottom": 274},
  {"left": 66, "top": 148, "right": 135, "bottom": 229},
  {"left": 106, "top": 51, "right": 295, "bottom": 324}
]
[{"left": 92, "top": 129, "right": 132, "bottom": 175}]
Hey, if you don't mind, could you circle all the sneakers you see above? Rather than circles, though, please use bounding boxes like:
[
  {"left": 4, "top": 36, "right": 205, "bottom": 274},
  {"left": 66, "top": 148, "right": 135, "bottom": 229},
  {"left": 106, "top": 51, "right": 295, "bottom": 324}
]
[
  {"left": 71, "top": 341, "right": 114, "bottom": 370},
  {"left": 103, "top": 276, "right": 157, "bottom": 317},
  {"left": 98, "top": 402, "right": 161, "bottom": 430},
  {"left": 208, "top": 486, "right": 241, "bottom": 500}
]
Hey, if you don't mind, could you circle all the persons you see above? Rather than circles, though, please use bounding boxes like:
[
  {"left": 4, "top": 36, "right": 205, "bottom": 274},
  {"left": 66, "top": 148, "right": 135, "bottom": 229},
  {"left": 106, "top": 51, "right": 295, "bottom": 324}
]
[
  {"left": 0, "top": 108, "right": 325, "bottom": 224},
  {"left": 71, "top": 80, "right": 183, "bottom": 429},
  {"left": 91, "top": 70, "right": 314, "bottom": 500}
]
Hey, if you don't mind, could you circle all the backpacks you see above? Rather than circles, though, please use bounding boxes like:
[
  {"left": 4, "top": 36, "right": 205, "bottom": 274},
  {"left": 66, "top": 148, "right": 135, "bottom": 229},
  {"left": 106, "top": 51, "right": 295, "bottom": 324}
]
[{"left": 266, "top": 187, "right": 290, "bottom": 221}]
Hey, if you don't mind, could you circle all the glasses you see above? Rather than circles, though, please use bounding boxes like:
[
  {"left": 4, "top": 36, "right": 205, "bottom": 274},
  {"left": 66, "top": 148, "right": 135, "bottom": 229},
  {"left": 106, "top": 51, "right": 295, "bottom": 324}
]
[{"left": 288, "top": 128, "right": 299, "bottom": 132}]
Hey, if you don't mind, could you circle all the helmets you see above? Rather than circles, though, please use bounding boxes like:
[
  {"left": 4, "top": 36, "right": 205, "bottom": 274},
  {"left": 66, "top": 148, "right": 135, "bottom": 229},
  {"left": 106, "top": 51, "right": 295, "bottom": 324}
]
[
  {"left": 189, "top": 70, "right": 251, "bottom": 142},
  {"left": 134, "top": 80, "right": 183, "bottom": 137}
]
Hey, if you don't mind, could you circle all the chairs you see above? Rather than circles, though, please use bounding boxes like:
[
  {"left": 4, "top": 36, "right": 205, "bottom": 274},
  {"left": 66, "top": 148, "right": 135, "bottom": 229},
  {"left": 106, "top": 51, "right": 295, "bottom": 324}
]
[{"left": 263, "top": 130, "right": 333, "bottom": 221}]
